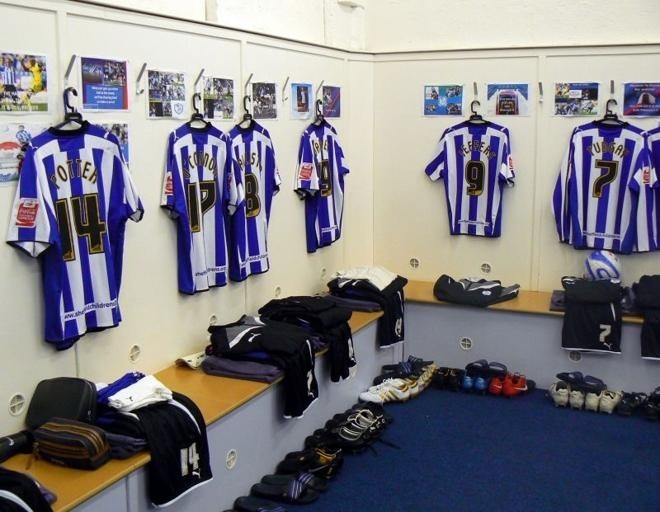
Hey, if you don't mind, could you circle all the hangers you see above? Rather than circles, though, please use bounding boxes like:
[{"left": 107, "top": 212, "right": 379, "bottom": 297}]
[
  {"left": 311, "top": 99, "right": 325, "bottom": 128},
  {"left": 466, "top": 100, "right": 488, "bottom": 124},
  {"left": 235, "top": 97, "right": 253, "bottom": 129},
  {"left": 186, "top": 91, "right": 208, "bottom": 129},
  {"left": 50, "top": 85, "right": 85, "bottom": 130},
  {"left": 592, "top": 98, "right": 625, "bottom": 126}
]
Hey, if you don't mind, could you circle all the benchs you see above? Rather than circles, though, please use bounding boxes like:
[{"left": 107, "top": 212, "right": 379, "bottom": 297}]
[
  {"left": 6, "top": 281, "right": 397, "bottom": 512},
  {"left": 404, "top": 280, "right": 660, "bottom": 395}
]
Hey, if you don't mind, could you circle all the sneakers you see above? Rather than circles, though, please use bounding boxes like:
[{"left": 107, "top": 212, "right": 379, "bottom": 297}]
[
  {"left": 359, "top": 354, "right": 535, "bottom": 403},
  {"left": 233, "top": 403, "right": 393, "bottom": 512},
  {"left": 548, "top": 371, "right": 660, "bottom": 422}
]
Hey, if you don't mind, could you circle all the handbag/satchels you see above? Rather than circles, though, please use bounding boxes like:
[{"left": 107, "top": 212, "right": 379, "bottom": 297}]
[
  {"left": 33, "top": 417, "right": 111, "bottom": 471},
  {"left": 26, "top": 378, "right": 98, "bottom": 428}
]
